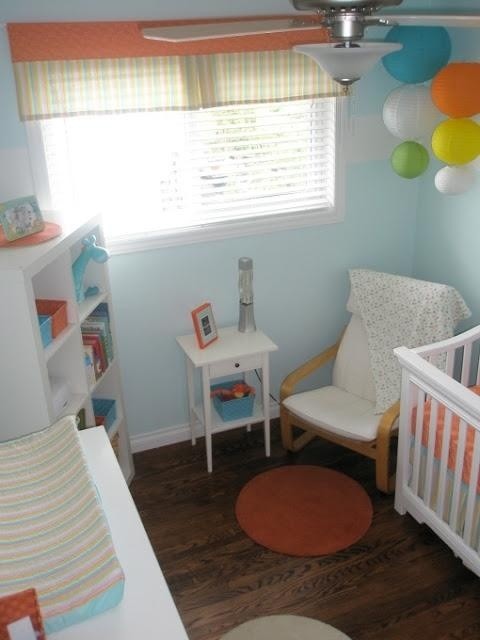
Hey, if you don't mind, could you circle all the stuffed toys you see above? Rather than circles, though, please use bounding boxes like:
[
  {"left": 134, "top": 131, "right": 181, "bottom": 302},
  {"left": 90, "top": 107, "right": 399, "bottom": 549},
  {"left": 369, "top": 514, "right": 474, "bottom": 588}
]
[{"left": 70, "top": 234, "right": 109, "bottom": 308}]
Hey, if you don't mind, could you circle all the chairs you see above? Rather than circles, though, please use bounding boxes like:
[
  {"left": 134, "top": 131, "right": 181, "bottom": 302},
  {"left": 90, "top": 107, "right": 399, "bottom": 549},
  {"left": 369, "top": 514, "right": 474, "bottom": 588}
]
[{"left": 278, "top": 269, "right": 471, "bottom": 494}]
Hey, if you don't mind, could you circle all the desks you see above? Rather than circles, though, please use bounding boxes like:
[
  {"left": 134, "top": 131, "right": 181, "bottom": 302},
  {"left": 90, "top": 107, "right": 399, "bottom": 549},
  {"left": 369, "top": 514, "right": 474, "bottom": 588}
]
[
  {"left": 176, "top": 324, "right": 279, "bottom": 473},
  {"left": 0, "top": 426, "right": 189, "bottom": 640}
]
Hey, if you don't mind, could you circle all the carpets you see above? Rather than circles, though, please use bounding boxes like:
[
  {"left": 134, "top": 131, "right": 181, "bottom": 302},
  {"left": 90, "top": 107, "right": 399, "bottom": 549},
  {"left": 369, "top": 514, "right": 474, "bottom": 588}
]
[
  {"left": 236, "top": 465, "right": 373, "bottom": 557},
  {"left": 218, "top": 614, "right": 352, "bottom": 640}
]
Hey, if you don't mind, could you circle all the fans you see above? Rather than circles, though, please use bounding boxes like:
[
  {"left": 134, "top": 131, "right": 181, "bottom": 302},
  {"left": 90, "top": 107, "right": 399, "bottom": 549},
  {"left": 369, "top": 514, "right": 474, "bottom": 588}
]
[{"left": 140, "top": 0, "right": 480, "bottom": 44}]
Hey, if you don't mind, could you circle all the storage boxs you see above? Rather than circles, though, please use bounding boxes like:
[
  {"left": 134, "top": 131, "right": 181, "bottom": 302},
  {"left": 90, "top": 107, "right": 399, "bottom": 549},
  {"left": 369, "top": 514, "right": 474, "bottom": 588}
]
[
  {"left": 93, "top": 398, "right": 116, "bottom": 434},
  {"left": 35, "top": 299, "right": 68, "bottom": 339},
  {"left": 39, "top": 313, "right": 52, "bottom": 347},
  {"left": 210, "top": 380, "right": 255, "bottom": 422}
]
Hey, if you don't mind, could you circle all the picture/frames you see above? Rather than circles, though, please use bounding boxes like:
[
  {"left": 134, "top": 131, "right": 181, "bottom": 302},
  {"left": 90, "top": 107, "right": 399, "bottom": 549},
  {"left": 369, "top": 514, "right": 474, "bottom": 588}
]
[
  {"left": 191, "top": 302, "right": 218, "bottom": 349},
  {"left": 0, "top": 196, "right": 45, "bottom": 241}
]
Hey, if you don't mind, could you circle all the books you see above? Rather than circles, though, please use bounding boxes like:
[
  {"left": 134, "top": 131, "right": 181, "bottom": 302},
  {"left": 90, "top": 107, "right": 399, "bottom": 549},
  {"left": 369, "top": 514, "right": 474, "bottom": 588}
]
[{"left": 80, "top": 303, "right": 114, "bottom": 388}]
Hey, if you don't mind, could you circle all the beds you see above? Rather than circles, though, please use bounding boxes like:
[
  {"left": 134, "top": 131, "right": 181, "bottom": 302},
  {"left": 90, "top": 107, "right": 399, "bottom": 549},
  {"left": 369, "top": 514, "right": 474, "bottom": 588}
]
[{"left": 393, "top": 324, "right": 479, "bottom": 577}]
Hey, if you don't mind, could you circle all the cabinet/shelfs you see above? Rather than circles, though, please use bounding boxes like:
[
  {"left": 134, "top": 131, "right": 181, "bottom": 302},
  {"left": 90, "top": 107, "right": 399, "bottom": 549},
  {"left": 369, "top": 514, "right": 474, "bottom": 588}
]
[{"left": 0, "top": 210, "right": 136, "bottom": 490}]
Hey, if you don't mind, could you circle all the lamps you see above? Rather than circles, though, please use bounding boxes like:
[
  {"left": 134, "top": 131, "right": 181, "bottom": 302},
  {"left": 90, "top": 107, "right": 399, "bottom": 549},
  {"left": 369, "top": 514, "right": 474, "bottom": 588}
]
[{"left": 290, "top": 40, "right": 403, "bottom": 84}]
